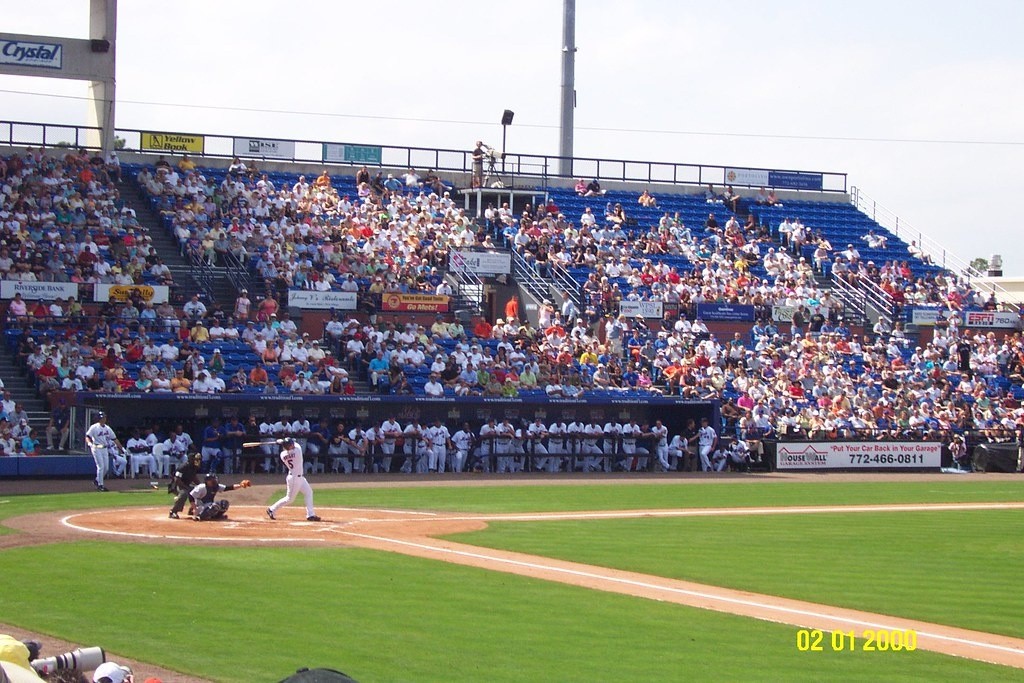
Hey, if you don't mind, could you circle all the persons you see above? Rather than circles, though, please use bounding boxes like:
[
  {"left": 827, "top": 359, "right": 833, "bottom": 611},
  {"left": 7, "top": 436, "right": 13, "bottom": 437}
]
[
  {"left": 0, "top": 634, "right": 162, "bottom": 683},
  {"left": 267, "top": 438, "right": 321, "bottom": 521},
  {"left": 0, "top": 288, "right": 156, "bottom": 492},
  {"left": 168, "top": 453, "right": 250, "bottom": 521},
  {"left": 140, "top": 141, "right": 1022, "bottom": 474},
  {"left": 0, "top": 145, "right": 177, "bottom": 297}
]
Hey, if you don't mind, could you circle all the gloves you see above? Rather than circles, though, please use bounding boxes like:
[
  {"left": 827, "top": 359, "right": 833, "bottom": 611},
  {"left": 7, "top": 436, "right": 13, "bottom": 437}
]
[
  {"left": 277, "top": 438, "right": 283, "bottom": 445},
  {"left": 88, "top": 442, "right": 92, "bottom": 447},
  {"left": 122, "top": 447, "right": 128, "bottom": 454}
]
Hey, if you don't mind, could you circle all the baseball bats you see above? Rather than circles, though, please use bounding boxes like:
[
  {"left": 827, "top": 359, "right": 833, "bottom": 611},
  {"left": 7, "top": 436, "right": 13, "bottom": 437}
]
[{"left": 242, "top": 441, "right": 276, "bottom": 447}]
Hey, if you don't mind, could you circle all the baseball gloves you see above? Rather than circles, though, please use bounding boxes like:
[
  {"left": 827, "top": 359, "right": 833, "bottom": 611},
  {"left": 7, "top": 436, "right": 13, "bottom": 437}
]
[{"left": 240, "top": 480, "right": 251, "bottom": 488}]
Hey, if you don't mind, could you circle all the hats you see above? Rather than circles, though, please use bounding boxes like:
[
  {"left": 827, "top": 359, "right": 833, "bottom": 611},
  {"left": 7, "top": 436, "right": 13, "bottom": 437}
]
[
  {"left": 0, "top": 634, "right": 39, "bottom": 677},
  {"left": 279, "top": 667, "right": 359, "bottom": 683},
  {"left": 19, "top": 639, "right": 42, "bottom": 650},
  {"left": 93, "top": 662, "right": 124, "bottom": 683},
  {"left": 15, "top": 441, "right": 23, "bottom": 449},
  {"left": 0, "top": 141, "right": 1024, "bottom": 438},
  {"left": 34, "top": 443, "right": 41, "bottom": 448}
]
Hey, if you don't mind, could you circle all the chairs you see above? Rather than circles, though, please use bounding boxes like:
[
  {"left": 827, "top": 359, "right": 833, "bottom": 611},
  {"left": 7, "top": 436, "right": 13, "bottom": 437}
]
[{"left": 0, "top": 160, "right": 1024, "bottom": 479}]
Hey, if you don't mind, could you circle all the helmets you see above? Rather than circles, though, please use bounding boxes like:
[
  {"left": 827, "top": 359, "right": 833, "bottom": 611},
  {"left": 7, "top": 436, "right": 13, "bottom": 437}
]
[
  {"left": 97, "top": 411, "right": 107, "bottom": 419},
  {"left": 188, "top": 453, "right": 200, "bottom": 466},
  {"left": 284, "top": 437, "right": 296, "bottom": 448},
  {"left": 204, "top": 473, "right": 219, "bottom": 486}
]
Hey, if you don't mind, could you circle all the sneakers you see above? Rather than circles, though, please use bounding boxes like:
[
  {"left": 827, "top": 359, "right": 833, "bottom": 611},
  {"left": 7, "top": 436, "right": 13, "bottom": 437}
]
[
  {"left": 170, "top": 510, "right": 179, "bottom": 519},
  {"left": 93, "top": 481, "right": 98, "bottom": 487},
  {"left": 307, "top": 515, "right": 321, "bottom": 521},
  {"left": 98, "top": 486, "right": 109, "bottom": 492},
  {"left": 115, "top": 458, "right": 763, "bottom": 484},
  {"left": 267, "top": 509, "right": 276, "bottom": 520}
]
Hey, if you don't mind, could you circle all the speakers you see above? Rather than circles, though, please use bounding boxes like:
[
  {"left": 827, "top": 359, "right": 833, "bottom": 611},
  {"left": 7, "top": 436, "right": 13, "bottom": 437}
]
[
  {"left": 502, "top": 110, "right": 514, "bottom": 125},
  {"left": 91, "top": 39, "right": 110, "bottom": 53}
]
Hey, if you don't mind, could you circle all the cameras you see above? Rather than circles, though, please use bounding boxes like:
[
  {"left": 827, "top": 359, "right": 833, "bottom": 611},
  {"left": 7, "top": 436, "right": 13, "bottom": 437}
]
[
  {"left": 119, "top": 666, "right": 133, "bottom": 682},
  {"left": 30, "top": 648, "right": 106, "bottom": 677}
]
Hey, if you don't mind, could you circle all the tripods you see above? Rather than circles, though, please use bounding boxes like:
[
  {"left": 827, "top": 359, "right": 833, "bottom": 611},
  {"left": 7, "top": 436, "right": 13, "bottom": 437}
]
[{"left": 482, "top": 157, "right": 501, "bottom": 188}]
